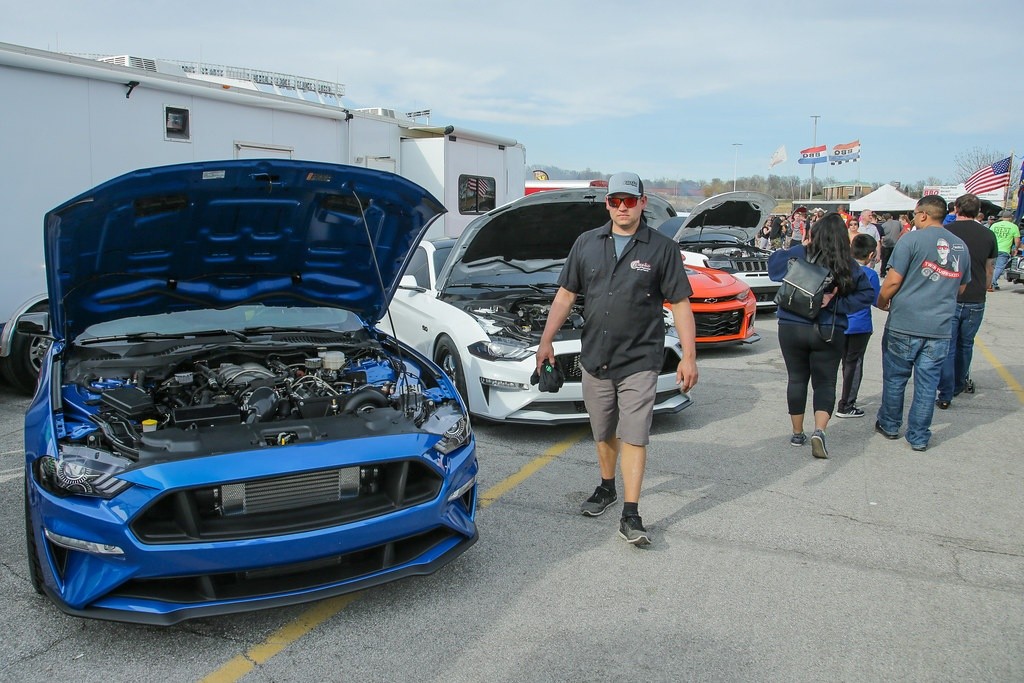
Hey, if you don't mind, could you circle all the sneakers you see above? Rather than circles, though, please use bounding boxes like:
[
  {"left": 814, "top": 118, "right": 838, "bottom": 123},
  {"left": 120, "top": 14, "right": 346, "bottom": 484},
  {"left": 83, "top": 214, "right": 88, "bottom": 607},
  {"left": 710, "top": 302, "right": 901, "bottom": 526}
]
[
  {"left": 618, "top": 510, "right": 651, "bottom": 545},
  {"left": 791, "top": 430, "right": 806, "bottom": 447},
  {"left": 811, "top": 429, "right": 828, "bottom": 459},
  {"left": 579, "top": 484, "right": 618, "bottom": 517},
  {"left": 835, "top": 403, "right": 864, "bottom": 418}
]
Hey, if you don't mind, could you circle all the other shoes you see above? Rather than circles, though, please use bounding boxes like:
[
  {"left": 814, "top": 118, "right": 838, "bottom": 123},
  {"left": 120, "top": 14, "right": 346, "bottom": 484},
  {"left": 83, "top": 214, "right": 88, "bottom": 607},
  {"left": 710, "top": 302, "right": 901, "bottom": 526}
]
[
  {"left": 876, "top": 420, "right": 899, "bottom": 439},
  {"left": 935, "top": 399, "right": 950, "bottom": 409},
  {"left": 965, "top": 379, "right": 974, "bottom": 393}
]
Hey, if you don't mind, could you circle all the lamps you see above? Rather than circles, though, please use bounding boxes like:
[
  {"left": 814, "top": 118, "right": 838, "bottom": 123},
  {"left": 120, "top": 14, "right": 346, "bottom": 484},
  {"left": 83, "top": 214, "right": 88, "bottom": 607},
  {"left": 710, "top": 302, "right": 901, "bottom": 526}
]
[{"left": 165, "top": 109, "right": 187, "bottom": 132}]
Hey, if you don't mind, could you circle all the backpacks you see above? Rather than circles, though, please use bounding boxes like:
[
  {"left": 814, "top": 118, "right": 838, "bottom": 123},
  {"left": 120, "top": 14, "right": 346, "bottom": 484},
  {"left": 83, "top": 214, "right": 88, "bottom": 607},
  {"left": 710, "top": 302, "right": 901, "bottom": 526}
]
[{"left": 773, "top": 247, "right": 839, "bottom": 343}]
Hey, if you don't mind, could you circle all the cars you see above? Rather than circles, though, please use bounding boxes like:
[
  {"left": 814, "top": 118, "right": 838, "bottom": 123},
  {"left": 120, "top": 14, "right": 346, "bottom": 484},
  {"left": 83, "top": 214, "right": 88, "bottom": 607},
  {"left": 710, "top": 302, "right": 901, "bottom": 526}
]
[
  {"left": 654, "top": 185, "right": 781, "bottom": 351},
  {"left": 0, "top": 291, "right": 50, "bottom": 394},
  {"left": 21, "top": 157, "right": 479, "bottom": 627},
  {"left": 371, "top": 187, "right": 693, "bottom": 424}
]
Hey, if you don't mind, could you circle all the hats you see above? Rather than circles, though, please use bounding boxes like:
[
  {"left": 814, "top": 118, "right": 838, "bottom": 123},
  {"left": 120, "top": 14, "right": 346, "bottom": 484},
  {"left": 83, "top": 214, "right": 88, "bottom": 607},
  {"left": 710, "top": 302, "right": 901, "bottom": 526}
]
[
  {"left": 988, "top": 216, "right": 996, "bottom": 220},
  {"left": 1002, "top": 211, "right": 1014, "bottom": 218},
  {"left": 607, "top": 172, "right": 644, "bottom": 196}
]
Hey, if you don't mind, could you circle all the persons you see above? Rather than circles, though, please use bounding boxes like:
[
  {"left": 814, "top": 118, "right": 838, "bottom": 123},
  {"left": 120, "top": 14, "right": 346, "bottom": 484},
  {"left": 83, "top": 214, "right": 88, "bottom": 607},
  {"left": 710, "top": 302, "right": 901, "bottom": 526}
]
[
  {"left": 535, "top": 172, "right": 699, "bottom": 544},
  {"left": 942, "top": 202, "right": 1024, "bottom": 231},
  {"left": 759, "top": 205, "right": 917, "bottom": 280},
  {"left": 768, "top": 214, "right": 876, "bottom": 458},
  {"left": 936, "top": 195, "right": 999, "bottom": 410},
  {"left": 875, "top": 194, "right": 972, "bottom": 451},
  {"left": 836, "top": 234, "right": 882, "bottom": 417},
  {"left": 989, "top": 211, "right": 1020, "bottom": 292}
]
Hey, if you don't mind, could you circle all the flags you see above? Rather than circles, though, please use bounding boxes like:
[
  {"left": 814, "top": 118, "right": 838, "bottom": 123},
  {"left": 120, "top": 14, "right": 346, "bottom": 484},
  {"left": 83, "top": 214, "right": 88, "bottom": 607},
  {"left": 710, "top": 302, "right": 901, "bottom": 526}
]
[
  {"left": 797, "top": 145, "right": 828, "bottom": 165},
  {"left": 964, "top": 154, "right": 1012, "bottom": 194},
  {"left": 830, "top": 138, "right": 861, "bottom": 167},
  {"left": 769, "top": 145, "right": 788, "bottom": 170}
]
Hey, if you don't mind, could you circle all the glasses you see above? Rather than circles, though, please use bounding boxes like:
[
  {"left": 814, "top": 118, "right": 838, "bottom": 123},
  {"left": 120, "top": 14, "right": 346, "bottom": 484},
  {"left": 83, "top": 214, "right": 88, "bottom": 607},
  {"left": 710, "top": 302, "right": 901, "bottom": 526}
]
[
  {"left": 912, "top": 211, "right": 930, "bottom": 218},
  {"left": 608, "top": 197, "right": 641, "bottom": 208}
]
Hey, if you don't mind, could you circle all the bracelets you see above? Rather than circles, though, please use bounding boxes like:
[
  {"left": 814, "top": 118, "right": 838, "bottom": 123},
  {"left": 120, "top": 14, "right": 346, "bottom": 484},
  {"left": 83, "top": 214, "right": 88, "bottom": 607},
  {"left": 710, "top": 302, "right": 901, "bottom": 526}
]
[{"left": 1014, "top": 247, "right": 1018, "bottom": 250}]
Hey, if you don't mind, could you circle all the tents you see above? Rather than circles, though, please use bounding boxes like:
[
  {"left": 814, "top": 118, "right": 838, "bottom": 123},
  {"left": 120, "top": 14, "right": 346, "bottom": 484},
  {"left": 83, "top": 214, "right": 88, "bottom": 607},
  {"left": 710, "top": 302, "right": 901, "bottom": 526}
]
[{"left": 849, "top": 183, "right": 918, "bottom": 216}]
